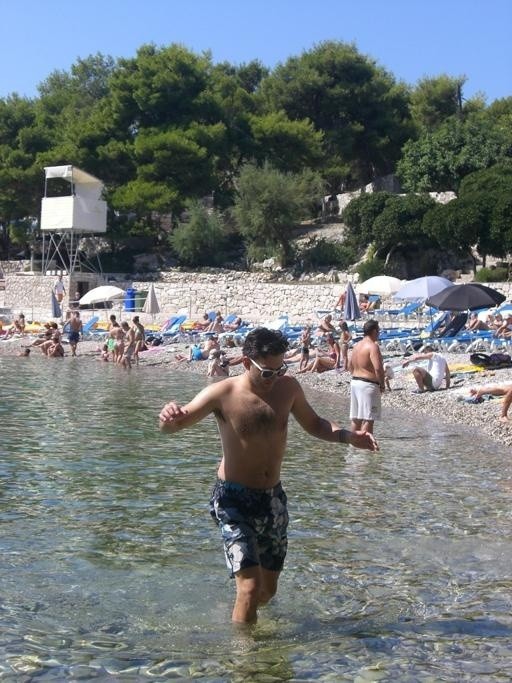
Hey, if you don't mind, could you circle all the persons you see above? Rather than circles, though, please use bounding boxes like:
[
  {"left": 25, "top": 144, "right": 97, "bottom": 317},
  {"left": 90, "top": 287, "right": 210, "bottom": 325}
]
[
  {"left": 347, "top": 319, "right": 386, "bottom": 438},
  {"left": 383, "top": 363, "right": 395, "bottom": 391},
  {"left": 159, "top": 328, "right": 379, "bottom": 629},
  {"left": 187, "top": 295, "right": 371, "bottom": 376},
  {"left": 438, "top": 311, "right": 512, "bottom": 339},
  {"left": 402, "top": 346, "right": 450, "bottom": 394},
  {"left": 1, "top": 275, "right": 148, "bottom": 369},
  {"left": 468, "top": 385, "right": 512, "bottom": 423}
]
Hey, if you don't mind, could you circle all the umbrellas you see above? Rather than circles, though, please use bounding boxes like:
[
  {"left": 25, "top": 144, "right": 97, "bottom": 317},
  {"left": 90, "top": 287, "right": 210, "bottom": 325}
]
[
  {"left": 142, "top": 282, "right": 160, "bottom": 325},
  {"left": 343, "top": 281, "right": 361, "bottom": 328},
  {"left": 392, "top": 276, "right": 456, "bottom": 315},
  {"left": 425, "top": 283, "right": 506, "bottom": 352},
  {"left": 354, "top": 275, "right": 404, "bottom": 311}
]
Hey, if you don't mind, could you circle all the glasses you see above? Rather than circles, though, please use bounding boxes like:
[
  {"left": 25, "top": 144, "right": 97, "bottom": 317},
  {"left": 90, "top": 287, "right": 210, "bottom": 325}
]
[{"left": 248, "top": 357, "right": 288, "bottom": 379}]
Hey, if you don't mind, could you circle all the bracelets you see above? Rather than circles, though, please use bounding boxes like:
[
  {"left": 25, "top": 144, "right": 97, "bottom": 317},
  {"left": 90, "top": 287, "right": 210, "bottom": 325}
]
[{"left": 380, "top": 385, "right": 385, "bottom": 389}]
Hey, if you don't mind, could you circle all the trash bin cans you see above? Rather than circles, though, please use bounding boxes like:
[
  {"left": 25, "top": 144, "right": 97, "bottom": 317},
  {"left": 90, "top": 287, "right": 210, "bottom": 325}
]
[
  {"left": 133, "top": 291, "right": 148, "bottom": 312},
  {"left": 125, "top": 288, "right": 138, "bottom": 311}
]
